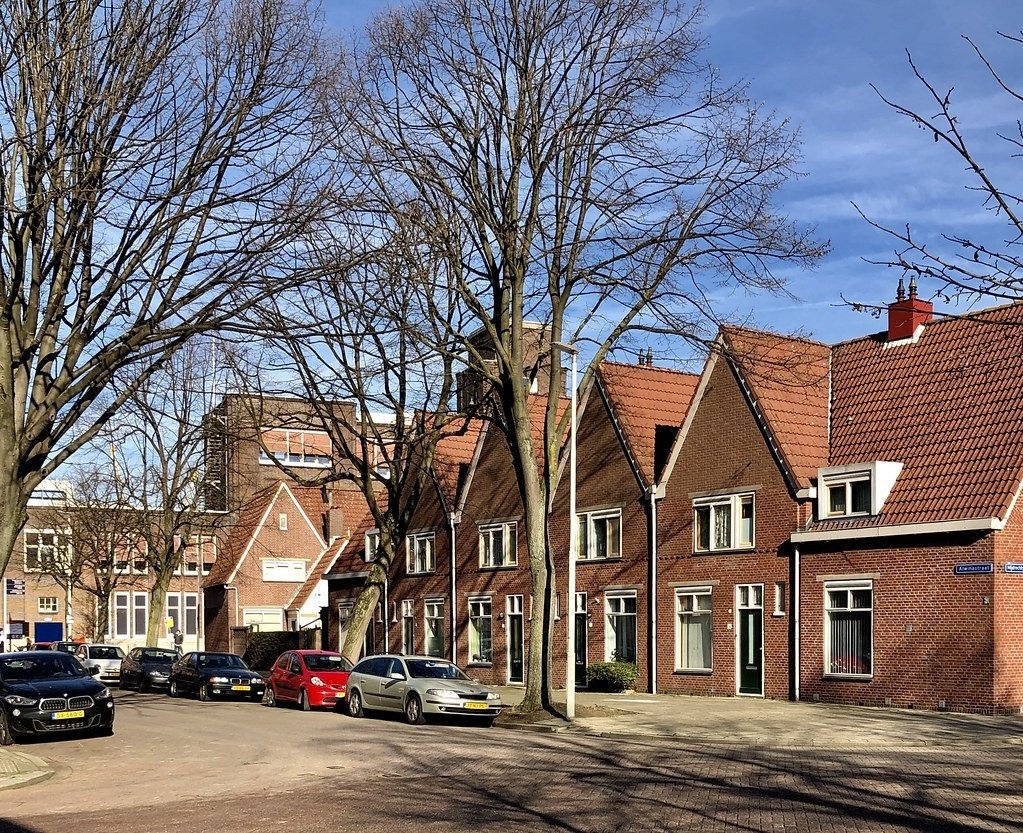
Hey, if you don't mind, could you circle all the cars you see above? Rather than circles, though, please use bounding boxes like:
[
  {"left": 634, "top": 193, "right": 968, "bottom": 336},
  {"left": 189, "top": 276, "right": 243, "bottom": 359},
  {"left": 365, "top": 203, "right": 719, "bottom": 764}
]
[
  {"left": 72, "top": 641, "right": 130, "bottom": 686},
  {"left": 168, "top": 651, "right": 266, "bottom": 702},
  {"left": 120, "top": 646, "right": 183, "bottom": 694},
  {"left": 0, "top": 650, "right": 115, "bottom": 745},
  {"left": 266, "top": 649, "right": 361, "bottom": 712},
  {"left": 27, "top": 641, "right": 88, "bottom": 659},
  {"left": 345, "top": 652, "right": 503, "bottom": 724}
]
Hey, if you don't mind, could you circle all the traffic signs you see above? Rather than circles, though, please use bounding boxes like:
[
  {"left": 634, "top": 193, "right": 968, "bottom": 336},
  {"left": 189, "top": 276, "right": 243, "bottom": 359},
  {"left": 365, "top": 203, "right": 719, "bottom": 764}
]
[
  {"left": 6, "top": 585, "right": 25, "bottom": 590},
  {"left": 6, "top": 590, "right": 25, "bottom": 595},
  {"left": 6, "top": 580, "right": 25, "bottom": 585}
]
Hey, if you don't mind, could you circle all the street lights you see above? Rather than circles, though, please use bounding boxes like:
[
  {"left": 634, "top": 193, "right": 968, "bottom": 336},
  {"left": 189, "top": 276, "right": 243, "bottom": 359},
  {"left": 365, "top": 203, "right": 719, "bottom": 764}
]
[{"left": 547, "top": 338, "right": 579, "bottom": 723}]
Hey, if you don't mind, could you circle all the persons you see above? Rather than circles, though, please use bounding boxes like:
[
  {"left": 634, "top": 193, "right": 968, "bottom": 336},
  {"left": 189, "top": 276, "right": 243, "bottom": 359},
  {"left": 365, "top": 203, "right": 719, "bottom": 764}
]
[
  {"left": 174, "top": 630, "right": 184, "bottom": 655},
  {"left": 27, "top": 638, "right": 32, "bottom": 651}
]
